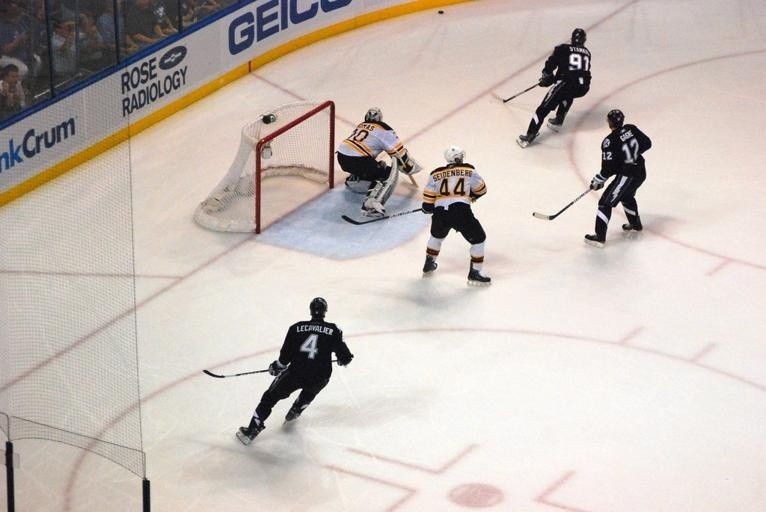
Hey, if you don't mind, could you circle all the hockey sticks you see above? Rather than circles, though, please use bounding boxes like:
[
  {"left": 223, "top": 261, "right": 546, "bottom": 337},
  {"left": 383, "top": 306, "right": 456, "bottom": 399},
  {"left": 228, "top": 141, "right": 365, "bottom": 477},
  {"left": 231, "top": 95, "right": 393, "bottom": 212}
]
[
  {"left": 491, "top": 82, "right": 541, "bottom": 103},
  {"left": 203, "top": 359, "right": 336, "bottom": 379},
  {"left": 531, "top": 188, "right": 592, "bottom": 219},
  {"left": 343, "top": 208, "right": 423, "bottom": 226}
]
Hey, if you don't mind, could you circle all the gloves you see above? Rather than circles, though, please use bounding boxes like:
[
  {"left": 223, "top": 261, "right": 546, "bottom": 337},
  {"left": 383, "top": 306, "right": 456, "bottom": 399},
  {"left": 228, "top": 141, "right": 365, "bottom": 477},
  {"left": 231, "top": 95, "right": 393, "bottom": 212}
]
[
  {"left": 538, "top": 71, "right": 557, "bottom": 89},
  {"left": 336, "top": 354, "right": 350, "bottom": 368},
  {"left": 267, "top": 359, "right": 288, "bottom": 376},
  {"left": 588, "top": 174, "right": 605, "bottom": 190}
]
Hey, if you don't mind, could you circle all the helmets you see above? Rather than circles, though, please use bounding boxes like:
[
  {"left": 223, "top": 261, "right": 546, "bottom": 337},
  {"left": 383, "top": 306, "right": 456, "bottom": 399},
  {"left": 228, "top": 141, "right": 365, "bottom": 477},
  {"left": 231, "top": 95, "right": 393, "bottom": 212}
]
[
  {"left": 606, "top": 109, "right": 624, "bottom": 129},
  {"left": 443, "top": 146, "right": 465, "bottom": 165},
  {"left": 570, "top": 28, "right": 586, "bottom": 48},
  {"left": 308, "top": 297, "right": 326, "bottom": 319},
  {"left": 363, "top": 106, "right": 383, "bottom": 123}
]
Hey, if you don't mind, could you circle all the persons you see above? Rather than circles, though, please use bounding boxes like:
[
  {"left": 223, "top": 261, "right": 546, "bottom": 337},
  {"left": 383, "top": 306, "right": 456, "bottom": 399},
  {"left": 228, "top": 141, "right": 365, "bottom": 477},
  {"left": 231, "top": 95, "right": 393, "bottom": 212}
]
[
  {"left": 585, "top": 110, "right": 652, "bottom": 248},
  {"left": 421, "top": 146, "right": 492, "bottom": 285},
  {"left": 1, "top": 1, "right": 225, "bottom": 117},
  {"left": 236, "top": 297, "right": 351, "bottom": 445},
  {"left": 336, "top": 106, "right": 415, "bottom": 217},
  {"left": 517, "top": 28, "right": 592, "bottom": 149}
]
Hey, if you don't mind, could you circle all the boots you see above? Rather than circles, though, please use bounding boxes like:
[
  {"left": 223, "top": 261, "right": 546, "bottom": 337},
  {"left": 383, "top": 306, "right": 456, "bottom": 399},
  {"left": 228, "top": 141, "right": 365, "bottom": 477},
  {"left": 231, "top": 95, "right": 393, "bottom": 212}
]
[
  {"left": 464, "top": 266, "right": 492, "bottom": 288},
  {"left": 515, "top": 127, "right": 540, "bottom": 151},
  {"left": 420, "top": 250, "right": 438, "bottom": 279},
  {"left": 283, "top": 395, "right": 307, "bottom": 430},
  {"left": 583, "top": 226, "right": 607, "bottom": 249},
  {"left": 343, "top": 170, "right": 360, "bottom": 192},
  {"left": 622, "top": 214, "right": 643, "bottom": 233},
  {"left": 234, "top": 410, "right": 264, "bottom": 447},
  {"left": 361, "top": 190, "right": 386, "bottom": 217},
  {"left": 546, "top": 110, "right": 566, "bottom": 134}
]
